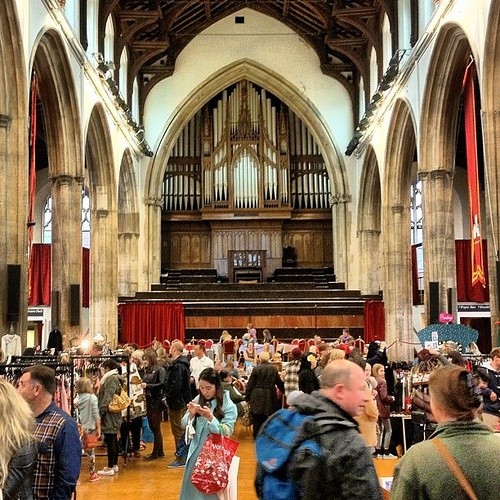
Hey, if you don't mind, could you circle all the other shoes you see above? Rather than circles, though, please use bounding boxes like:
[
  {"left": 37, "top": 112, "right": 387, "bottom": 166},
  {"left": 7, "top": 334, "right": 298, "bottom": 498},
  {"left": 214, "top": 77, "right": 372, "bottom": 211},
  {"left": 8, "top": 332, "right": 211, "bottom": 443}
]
[
  {"left": 377, "top": 454, "right": 383, "bottom": 459},
  {"left": 143, "top": 455, "right": 159, "bottom": 460},
  {"left": 383, "top": 453, "right": 398, "bottom": 460},
  {"left": 134, "top": 449, "right": 141, "bottom": 458},
  {"left": 88, "top": 472, "right": 101, "bottom": 482},
  {"left": 167, "top": 459, "right": 186, "bottom": 469},
  {"left": 104, "top": 465, "right": 119, "bottom": 472},
  {"left": 97, "top": 469, "right": 114, "bottom": 476}
]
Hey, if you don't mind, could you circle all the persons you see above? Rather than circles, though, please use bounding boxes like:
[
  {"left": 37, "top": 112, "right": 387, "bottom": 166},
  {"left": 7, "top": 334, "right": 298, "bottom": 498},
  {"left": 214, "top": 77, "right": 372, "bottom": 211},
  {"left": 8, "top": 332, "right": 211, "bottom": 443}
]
[
  {"left": 72, "top": 377, "right": 102, "bottom": 484},
  {"left": 1, "top": 380, "right": 37, "bottom": 500},
  {"left": 17, "top": 364, "right": 83, "bottom": 500},
  {"left": 176, "top": 367, "right": 238, "bottom": 500},
  {"left": 20, "top": 323, "right": 500, "bottom": 468},
  {"left": 96, "top": 359, "right": 124, "bottom": 476},
  {"left": 387, "top": 364, "right": 500, "bottom": 499},
  {"left": 286, "top": 359, "right": 384, "bottom": 500}
]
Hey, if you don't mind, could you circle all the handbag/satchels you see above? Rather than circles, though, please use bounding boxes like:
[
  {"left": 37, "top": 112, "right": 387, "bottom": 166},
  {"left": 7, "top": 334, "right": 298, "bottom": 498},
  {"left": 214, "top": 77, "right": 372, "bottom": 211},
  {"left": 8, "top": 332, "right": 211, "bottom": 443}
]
[
  {"left": 108, "top": 375, "right": 131, "bottom": 412},
  {"left": 217, "top": 424, "right": 240, "bottom": 500},
  {"left": 191, "top": 423, "right": 240, "bottom": 494},
  {"left": 85, "top": 433, "right": 99, "bottom": 448}
]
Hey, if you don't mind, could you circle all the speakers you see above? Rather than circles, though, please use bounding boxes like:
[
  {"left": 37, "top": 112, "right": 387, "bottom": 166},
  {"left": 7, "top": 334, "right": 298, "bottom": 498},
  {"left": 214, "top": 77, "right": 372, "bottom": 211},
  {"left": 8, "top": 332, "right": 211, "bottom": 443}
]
[
  {"left": 68, "top": 284, "right": 81, "bottom": 326},
  {"left": 429, "top": 281, "right": 440, "bottom": 325},
  {"left": 7, "top": 264, "right": 21, "bottom": 322}
]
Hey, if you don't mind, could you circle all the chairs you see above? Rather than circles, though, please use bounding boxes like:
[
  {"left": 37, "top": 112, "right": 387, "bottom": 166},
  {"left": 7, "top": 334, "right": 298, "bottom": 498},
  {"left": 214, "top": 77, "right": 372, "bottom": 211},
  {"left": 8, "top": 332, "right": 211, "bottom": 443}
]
[{"left": 163, "top": 336, "right": 365, "bottom": 362}]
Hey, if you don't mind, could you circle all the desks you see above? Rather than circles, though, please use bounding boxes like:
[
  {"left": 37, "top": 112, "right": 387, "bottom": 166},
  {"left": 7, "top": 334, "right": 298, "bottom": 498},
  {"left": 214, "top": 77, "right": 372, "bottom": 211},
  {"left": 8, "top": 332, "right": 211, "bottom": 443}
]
[
  {"left": 283, "top": 344, "right": 299, "bottom": 362},
  {"left": 238, "top": 344, "right": 274, "bottom": 366},
  {"left": 390, "top": 413, "right": 411, "bottom": 455},
  {"left": 278, "top": 343, "right": 286, "bottom": 362},
  {"left": 182, "top": 347, "right": 214, "bottom": 361}
]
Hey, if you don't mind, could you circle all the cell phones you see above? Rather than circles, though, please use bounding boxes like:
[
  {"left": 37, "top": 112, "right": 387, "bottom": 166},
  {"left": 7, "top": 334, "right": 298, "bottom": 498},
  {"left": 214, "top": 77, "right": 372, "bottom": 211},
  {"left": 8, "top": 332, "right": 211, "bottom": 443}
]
[{"left": 193, "top": 404, "right": 201, "bottom": 408}]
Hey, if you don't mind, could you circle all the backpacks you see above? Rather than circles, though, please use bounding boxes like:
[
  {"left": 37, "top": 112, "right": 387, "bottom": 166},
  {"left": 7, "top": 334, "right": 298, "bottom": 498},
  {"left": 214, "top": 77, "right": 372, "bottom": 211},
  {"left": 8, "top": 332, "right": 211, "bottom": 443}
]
[{"left": 253, "top": 406, "right": 358, "bottom": 500}]
